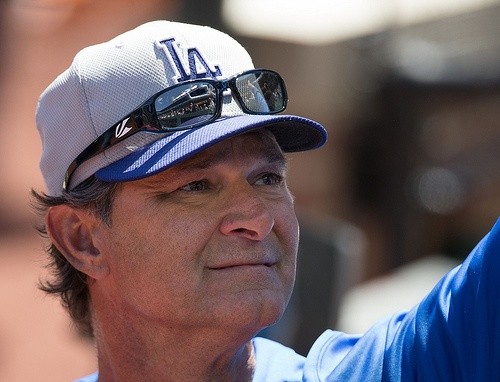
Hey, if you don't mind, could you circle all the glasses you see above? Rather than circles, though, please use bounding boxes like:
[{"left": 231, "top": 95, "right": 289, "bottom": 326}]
[{"left": 62, "top": 68, "right": 289, "bottom": 191}]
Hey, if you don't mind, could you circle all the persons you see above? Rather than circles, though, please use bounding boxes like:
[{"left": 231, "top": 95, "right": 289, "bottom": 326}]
[{"left": 31, "top": 20, "right": 500, "bottom": 382}]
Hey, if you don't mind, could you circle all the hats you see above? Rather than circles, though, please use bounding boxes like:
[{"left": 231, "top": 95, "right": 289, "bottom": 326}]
[{"left": 36, "top": 20, "right": 327, "bottom": 200}]
